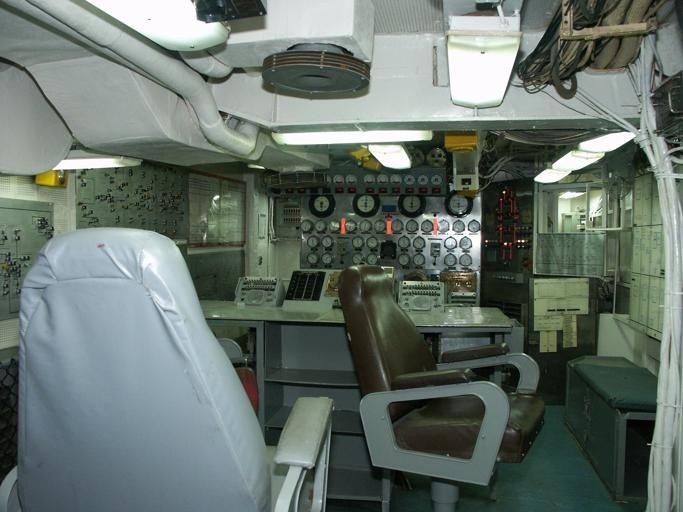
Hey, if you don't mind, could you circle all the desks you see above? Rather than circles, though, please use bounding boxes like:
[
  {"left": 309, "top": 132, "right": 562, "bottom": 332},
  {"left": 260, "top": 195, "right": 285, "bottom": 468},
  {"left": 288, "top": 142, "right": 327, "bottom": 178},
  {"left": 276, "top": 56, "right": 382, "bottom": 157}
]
[{"left": 199, "top": 298, "right": 514, "bottom": 512}]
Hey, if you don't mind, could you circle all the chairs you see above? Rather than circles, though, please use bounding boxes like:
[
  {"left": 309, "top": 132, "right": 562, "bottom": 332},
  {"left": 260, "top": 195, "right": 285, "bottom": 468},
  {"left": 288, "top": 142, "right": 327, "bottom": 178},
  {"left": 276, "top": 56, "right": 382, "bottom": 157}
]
[
  {"left": 336, "top": 264, "right": 543, "bottom": 512},
  {"left": 0, "top": 228, "right": 336, "bottom": 512}
]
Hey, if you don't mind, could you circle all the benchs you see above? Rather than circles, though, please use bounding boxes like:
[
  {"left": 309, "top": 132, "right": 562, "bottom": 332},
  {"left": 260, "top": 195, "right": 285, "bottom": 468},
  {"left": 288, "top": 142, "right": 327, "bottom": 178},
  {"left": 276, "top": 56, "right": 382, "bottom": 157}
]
[{"left": 567, "top": 354, "right": 659, "bottom": 506}]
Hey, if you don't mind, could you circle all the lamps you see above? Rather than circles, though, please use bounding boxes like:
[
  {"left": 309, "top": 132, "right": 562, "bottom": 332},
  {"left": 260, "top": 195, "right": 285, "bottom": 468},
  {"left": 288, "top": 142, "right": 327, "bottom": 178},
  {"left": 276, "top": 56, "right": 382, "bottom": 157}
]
[
  {"left": 532, "top": 129, "right": 635, "bottom": 184},
  {"left": 270, "top": 130, "right": 432, "bottom": 146},
  {"left": 366, "top": 142, "right": 411, "bottom": 170},
  {"left": 49, "top": 149, "right": 142, "bottom": 171},
  {"left": 444, "top": 16, "right": 522, "bottom": 109}
]
